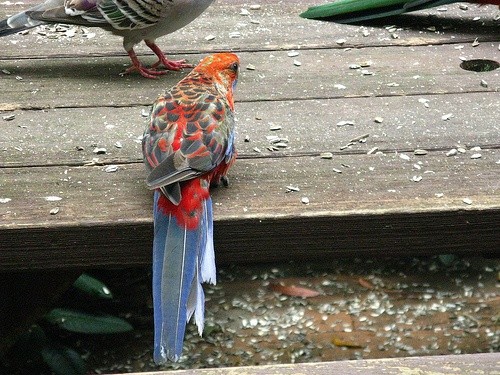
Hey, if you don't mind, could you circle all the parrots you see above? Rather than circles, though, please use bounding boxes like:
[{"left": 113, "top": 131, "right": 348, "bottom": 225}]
[{"left": 142, "top": 53, "right": 240, "bottom": 366}]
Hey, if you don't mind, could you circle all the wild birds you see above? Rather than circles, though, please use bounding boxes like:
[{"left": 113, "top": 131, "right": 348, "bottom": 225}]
[
  {"left": 298, "top": 0, "right": 500, "bottom": 24},
  {"left": 0, "top": 0, "right": 216, "bottom": 79}
]
